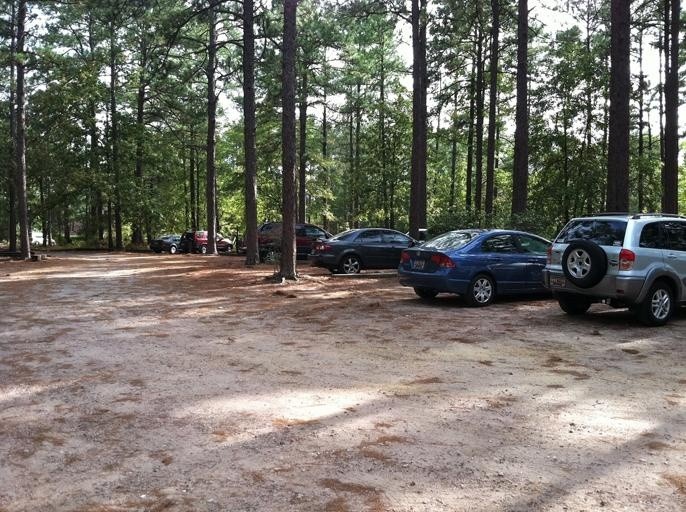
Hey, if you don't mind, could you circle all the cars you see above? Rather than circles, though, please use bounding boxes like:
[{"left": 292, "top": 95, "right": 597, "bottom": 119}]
[
  {"left": 149, "top": 234, "right": 188, "bottom": 255},
  {"left": 399, "top": 227, "right": 551, "bottom": 302},
  {"left": 243, "top": 218, "right": 337, "bottom": 261},
  {"left": 307, "top": 226, "right": 425, "bottom": 273}
]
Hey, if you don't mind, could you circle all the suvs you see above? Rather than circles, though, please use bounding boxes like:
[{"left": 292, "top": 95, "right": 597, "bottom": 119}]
[
  {"left": 542, "top": 212, "right": 686, "bottom": 326},
  {"left": 180, "top": 230, "right": 234, "bottom": 254}
]
